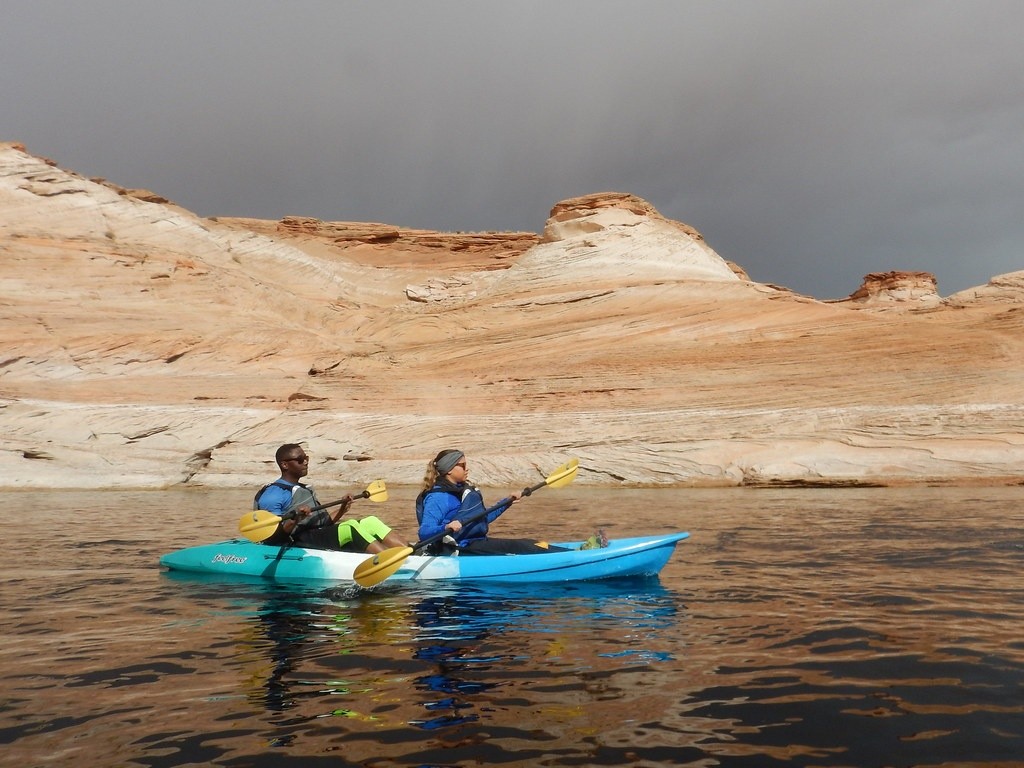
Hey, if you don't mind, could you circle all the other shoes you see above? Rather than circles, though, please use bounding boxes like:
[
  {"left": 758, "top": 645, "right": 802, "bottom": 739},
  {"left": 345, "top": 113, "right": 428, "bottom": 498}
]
[
  {"left": 599, "top": 527, "right": 608, "bottom": 548},
  {"left": 578, "top": 530, "right": 604, "bottom": 550},
  {"left": 414, "top": 545, "right": 438, "bottom": 556}
]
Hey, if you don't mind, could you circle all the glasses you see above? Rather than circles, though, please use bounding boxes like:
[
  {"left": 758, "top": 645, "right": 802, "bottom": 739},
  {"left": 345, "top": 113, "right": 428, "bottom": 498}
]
[
  {"left": 283, "top": 456, "right": 309, "bottom": 464},
  {"left": 456, "top": 462, "right": 467, "bottom": 470}
]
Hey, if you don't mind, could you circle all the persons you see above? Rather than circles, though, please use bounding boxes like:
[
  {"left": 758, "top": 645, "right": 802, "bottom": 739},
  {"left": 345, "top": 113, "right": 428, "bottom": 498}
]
[
  {"left": 258, "top": 443, "right": 429, "bottom": 557},
  {"left": 418, "top": 449, "right": 608, "bottom": 556}
]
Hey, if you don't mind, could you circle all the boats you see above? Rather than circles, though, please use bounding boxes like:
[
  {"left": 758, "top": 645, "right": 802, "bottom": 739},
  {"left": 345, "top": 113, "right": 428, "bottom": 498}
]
[{"left": 158, "top": 529, "right": 694, "bottom": 583}]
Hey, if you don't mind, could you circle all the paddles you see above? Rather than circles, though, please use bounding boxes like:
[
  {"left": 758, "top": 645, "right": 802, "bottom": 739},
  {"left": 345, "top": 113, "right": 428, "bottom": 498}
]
[
  {"left": 352, "top": 457, "right": 580, "bottom": 588},
  {"left": 237, "top": 479, "right": 389, "bottom": 543}
]
